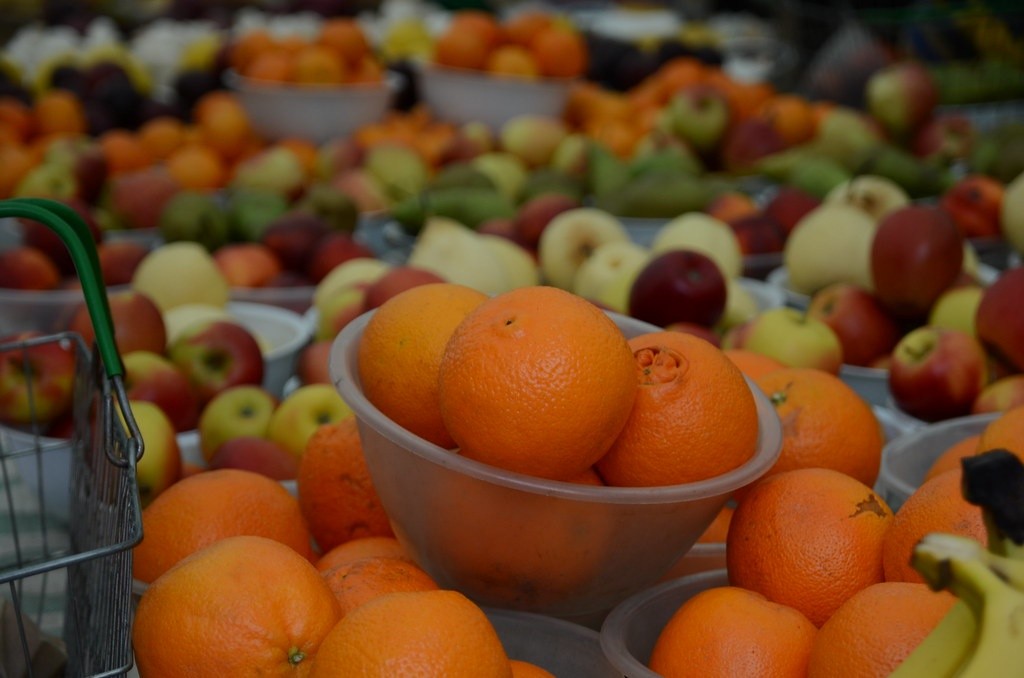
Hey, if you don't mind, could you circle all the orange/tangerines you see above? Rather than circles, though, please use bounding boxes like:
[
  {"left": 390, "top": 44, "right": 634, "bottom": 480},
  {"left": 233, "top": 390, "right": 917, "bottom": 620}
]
[
  {"left": 131, "top": 281, "right": 1024, "bottom": 677},
  {"left": 2, "top": 11, "right": 855, "bottom": 179}
]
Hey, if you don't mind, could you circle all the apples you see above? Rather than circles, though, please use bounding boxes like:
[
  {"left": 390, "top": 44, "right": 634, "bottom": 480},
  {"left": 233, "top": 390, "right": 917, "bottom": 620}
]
[{"left": 1, "top": 65, "right": 1024, "bottom": 495}]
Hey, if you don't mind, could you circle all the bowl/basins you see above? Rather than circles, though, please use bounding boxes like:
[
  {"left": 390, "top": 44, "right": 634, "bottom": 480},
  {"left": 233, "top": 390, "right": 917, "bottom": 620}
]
[
  {"left": 611, "top": 213, "right": 671, "bottom": 247},
  {"left": 838, "top": 360, "right": 1006, "bottom": 510},
  {"left": 600, "top": 566, "right": 729, "bottom": 678},
  {"left": 679, "top": 534, "right": 729, "bottom": 561},
  {"left": 330, "top": 303, "right": 784, "bottom": 616},
  {"left": 414, "top": 66, "right": 572, "bottom": 131},
  {"left": 225, "top": 68, "right": 403, "bottom": 146},
  {"left": 964, "top": 261, "right": 998, "bottom": 285},
  {"left": 0, "top": 213, "right": 318, "bottom": 678},
  {"left": 733, "top": 248, "right": 813, "bottom": 312},
  {"left": 478, "top": 606, "right": 622, "bottom": 678}
]
